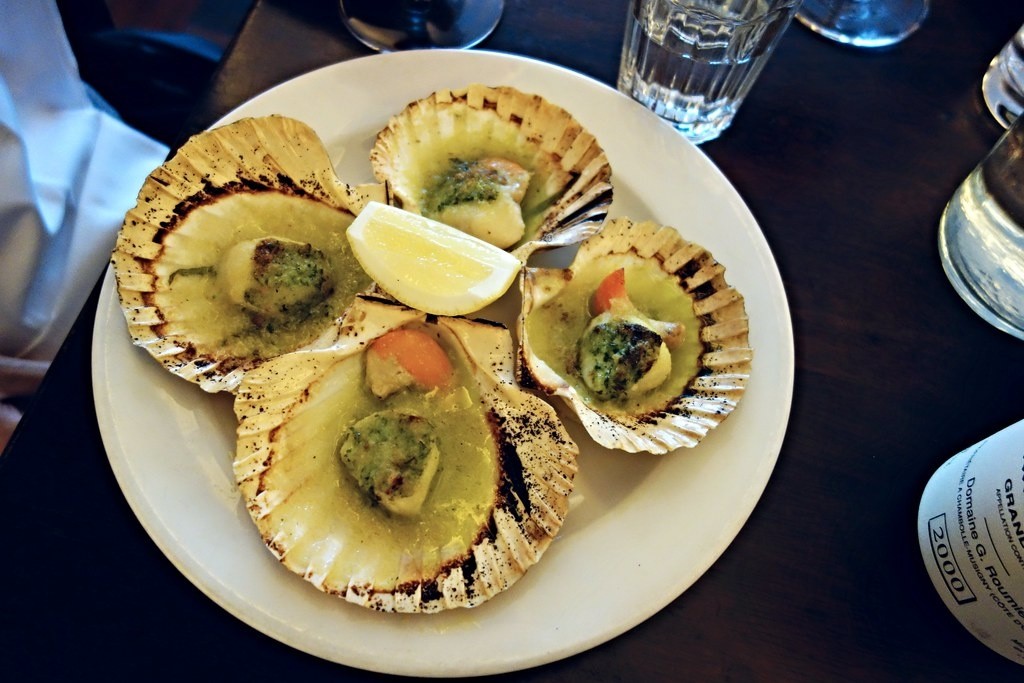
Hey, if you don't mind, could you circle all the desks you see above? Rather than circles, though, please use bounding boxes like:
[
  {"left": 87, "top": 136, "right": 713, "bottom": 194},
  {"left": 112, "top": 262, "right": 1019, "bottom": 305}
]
[{"left": 0, "top": 0, "right": 1024, "bottom": 683}]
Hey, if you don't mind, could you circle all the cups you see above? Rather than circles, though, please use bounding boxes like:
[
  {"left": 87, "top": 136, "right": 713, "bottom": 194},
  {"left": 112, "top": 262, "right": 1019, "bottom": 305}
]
[
  {"left": 615, "top": 1, "right": 802, "bottom": 146},
  {"left": 982, "top": 26, "right": 1024, "bottom": 128},
  {"left": 936, "top": 114, "right": 1024, "bottom": 342}
]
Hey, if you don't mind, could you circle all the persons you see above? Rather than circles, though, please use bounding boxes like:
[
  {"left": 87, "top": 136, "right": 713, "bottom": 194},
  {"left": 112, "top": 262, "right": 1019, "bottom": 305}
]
[{"left": 0, "top": 0, "right": 169, "bottom": 452}]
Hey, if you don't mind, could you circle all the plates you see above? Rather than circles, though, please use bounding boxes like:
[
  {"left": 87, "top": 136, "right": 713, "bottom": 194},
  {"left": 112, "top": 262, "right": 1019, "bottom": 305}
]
[{"left": 89, "top": 43, "right": 797, "bottom": 681}]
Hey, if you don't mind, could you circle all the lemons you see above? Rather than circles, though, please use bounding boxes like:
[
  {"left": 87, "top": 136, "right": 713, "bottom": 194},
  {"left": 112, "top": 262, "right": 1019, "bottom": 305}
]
[{"left": 345, "top": 200, "right": 522, "bottom": 318}]
